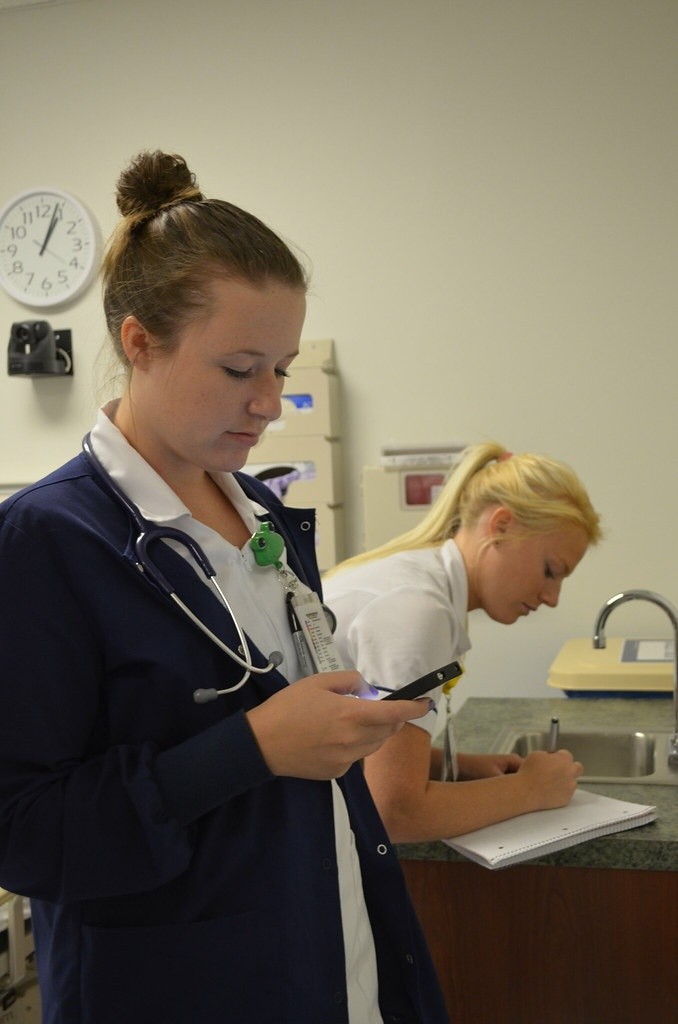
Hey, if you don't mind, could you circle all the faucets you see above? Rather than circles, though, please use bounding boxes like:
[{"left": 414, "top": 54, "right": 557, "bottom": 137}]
[{"left": 592, "top": 588, "right": 678, "bottom": 772}]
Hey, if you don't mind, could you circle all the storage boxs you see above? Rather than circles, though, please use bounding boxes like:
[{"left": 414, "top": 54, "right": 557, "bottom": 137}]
[{"left": 238, "top": 339, "right": 348, "bottom": 572}]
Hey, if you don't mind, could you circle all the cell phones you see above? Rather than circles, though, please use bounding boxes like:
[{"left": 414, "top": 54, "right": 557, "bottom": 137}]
[{"left": 381, "top": 661, "right": 463, "bottom": 702}]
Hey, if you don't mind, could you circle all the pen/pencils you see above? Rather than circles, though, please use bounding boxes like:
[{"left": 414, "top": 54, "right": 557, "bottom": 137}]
[{"left": 545, "top": 716, "right": 563, "bottom": 755}]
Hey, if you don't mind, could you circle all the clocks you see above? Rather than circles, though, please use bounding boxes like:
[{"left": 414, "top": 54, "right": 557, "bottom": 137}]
[{"left": 0, "top": 186, "right": 102, "bottom": 309}]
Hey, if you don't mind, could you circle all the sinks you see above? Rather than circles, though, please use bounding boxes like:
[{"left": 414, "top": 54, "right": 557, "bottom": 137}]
[{"left": 484, "top": 721, "right": 678, "bottom": 785}]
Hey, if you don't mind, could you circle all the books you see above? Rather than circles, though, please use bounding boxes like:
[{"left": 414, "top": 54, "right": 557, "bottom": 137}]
[{"left": 442, "top": 786, "right": 659, "bottom": 873}]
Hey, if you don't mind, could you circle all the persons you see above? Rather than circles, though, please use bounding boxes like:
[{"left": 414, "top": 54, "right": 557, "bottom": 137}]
[
  {"left": 0, "top": 149, "right": 448, "bottom": 1024},
  {"left": 320, "top": 442, "right": 604, "bottom": 845}
]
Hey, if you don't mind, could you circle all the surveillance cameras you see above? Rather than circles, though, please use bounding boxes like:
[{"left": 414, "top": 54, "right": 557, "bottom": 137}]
[{"left": 12, "top": 320, "right": 49, "bottom": 344}]
